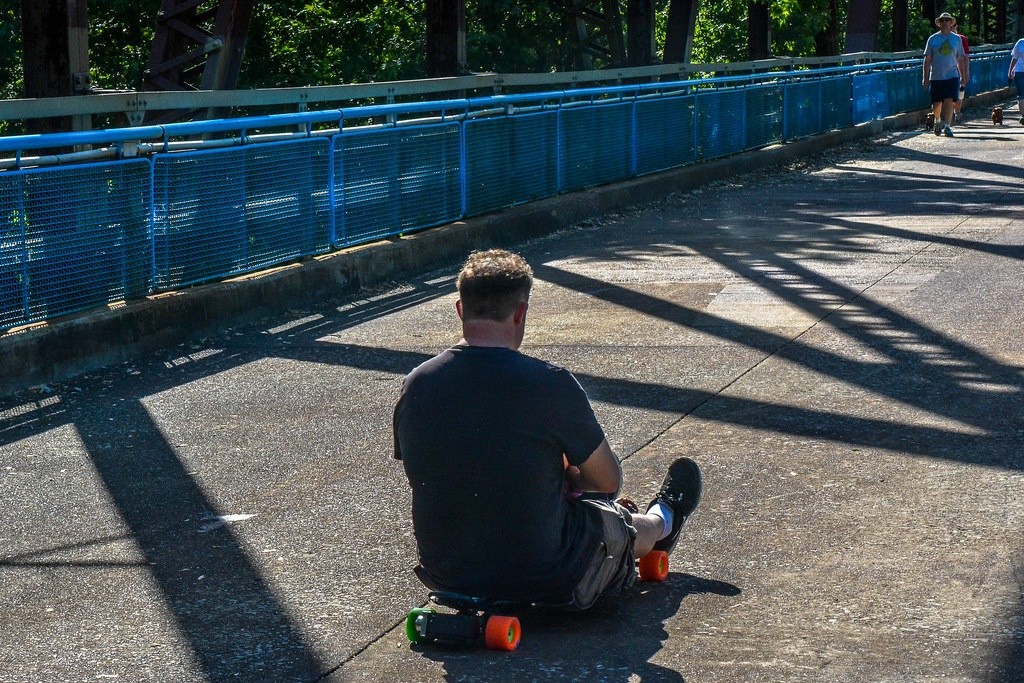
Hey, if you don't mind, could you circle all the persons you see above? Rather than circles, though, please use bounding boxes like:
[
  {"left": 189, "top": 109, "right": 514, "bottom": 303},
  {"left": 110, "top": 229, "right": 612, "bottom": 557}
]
[
  {"left": 394, "top": 248, "right": 701, "bottom": 617},
  {"left": 1008, "top": 38, "right": 1024, "bottom": 125},
  {"left": 922, "top": 12, "right": 969, "bottom": 138}
]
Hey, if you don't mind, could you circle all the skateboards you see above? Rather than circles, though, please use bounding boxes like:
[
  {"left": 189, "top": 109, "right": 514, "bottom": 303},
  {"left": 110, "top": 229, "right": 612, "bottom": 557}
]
[{"left": 402, "top": 537, "right": 675, "bottom": 655}]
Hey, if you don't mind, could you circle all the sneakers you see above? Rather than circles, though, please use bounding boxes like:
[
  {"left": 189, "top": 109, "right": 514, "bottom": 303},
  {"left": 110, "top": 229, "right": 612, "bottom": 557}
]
[
  {"left": 944, "top": 127, "right": 954, "bottom": 137},
  {"left": 954, "top": 110, "right": 962, "bottom": 125},
  {"left": 933, "top": 117, "right": 942, "bottom": 136},
  {"left": 1019, "top": 114, "right": 1024, "bottom": 125},
  {"left": 646, "top": 457, "right": 703, "bottom": 555}
]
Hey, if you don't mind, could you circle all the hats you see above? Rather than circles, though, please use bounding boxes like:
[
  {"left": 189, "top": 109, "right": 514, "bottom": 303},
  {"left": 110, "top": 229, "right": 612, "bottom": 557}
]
[{"left": 935, "top": 12, "right": 956, "bottom": 27}]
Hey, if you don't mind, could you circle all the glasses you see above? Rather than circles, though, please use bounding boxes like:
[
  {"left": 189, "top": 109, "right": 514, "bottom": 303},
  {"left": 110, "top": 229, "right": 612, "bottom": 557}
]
[{"left": 938, "top": 19, "right": 950, "bottom": 22}]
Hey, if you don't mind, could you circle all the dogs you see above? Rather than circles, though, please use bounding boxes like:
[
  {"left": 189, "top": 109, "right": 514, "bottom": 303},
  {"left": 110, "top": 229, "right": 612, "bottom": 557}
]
[
  {"left": 991, "top": 106, "right": 1004, "bottom": 126},
  {"left": 925, "top": 112, "right": 935, "bottom": 131}
]
